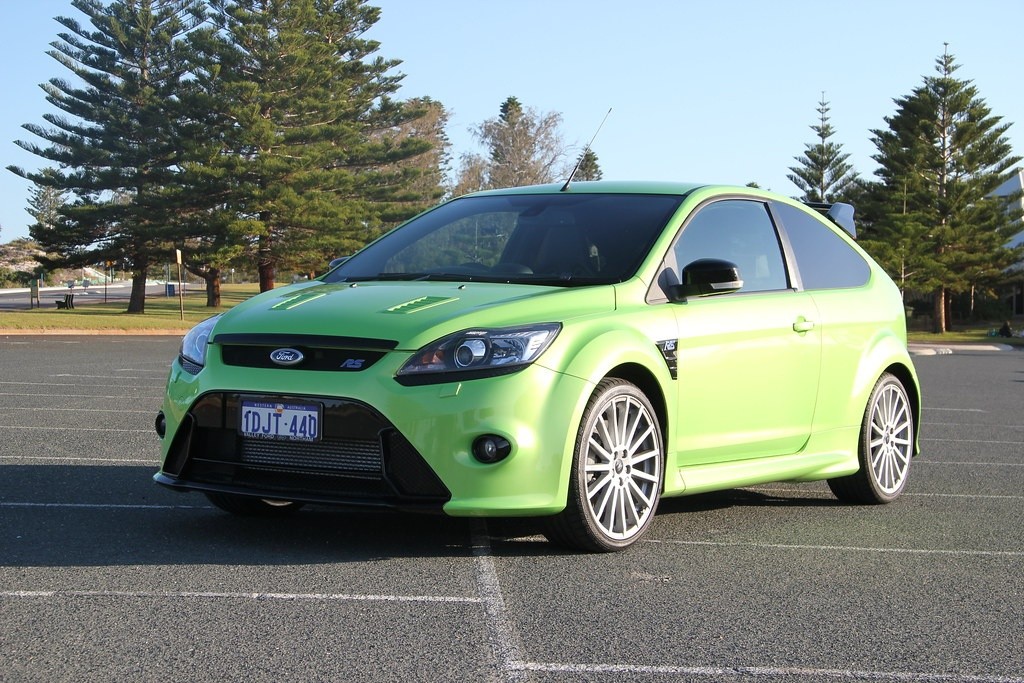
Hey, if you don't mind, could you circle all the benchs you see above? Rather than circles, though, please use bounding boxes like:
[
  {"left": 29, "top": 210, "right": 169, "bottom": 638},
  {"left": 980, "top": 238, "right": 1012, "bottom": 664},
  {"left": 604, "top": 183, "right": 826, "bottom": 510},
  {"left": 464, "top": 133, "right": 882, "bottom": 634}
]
[{"left": 55, "top": 294, "right": 74, "bottom": 309}]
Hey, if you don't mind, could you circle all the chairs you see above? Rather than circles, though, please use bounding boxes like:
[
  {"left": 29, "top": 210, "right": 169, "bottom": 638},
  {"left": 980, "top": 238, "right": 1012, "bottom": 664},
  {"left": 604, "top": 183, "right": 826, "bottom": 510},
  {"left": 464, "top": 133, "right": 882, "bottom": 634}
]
[{"left": 532, "top": 224, "right": 601, "bottom": 282}]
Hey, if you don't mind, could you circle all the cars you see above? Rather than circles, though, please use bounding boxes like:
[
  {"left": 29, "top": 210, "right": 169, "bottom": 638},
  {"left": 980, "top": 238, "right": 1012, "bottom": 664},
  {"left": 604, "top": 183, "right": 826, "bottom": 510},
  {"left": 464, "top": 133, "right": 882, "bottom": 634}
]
[{"left": 153, "top": 180, "right": 920, "bottom": 553}]
